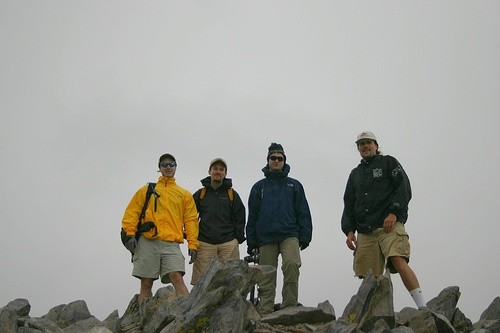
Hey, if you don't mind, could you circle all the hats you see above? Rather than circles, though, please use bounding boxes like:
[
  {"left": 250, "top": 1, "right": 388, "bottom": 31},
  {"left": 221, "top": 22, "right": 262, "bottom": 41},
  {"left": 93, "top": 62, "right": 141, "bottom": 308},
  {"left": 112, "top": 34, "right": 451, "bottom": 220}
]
[
  {"left": 355, "top": 132, "right": 376, "bottom": 143},
  {"left": 209, "top": 158, "right": 227, "bottom": 170},
  {"left": 267, "top": 142, "right": 286, "bottom": 161},
  {"left": 159, "top": 154, "right": 178, "bottom": 166}
]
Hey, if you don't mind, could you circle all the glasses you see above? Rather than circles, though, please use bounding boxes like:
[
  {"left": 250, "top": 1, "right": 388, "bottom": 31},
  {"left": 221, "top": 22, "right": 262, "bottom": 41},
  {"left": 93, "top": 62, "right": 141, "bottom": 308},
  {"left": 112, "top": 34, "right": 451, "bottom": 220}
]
[
  {"left": 160, "top": 162, "right": 174, "bottom": 167},
  {"left": 269, "top": 156, "right": 284, "bottom": 161}
]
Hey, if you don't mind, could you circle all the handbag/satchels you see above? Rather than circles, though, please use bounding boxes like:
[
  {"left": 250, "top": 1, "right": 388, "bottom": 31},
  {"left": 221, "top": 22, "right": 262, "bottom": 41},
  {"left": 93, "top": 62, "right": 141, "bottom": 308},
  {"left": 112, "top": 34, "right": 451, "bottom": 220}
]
[{"left": 120, "top": 227, "right": 137, "bottom": 246}]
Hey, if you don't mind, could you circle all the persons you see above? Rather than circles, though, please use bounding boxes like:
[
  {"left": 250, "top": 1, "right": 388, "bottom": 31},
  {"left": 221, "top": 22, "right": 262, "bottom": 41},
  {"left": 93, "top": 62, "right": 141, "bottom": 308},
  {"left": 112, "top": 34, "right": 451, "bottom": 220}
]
[
  {"left": 121, "top": 153, "right": 199, "bottom": 305},
  {"left": 246, "top": 142, "right": 312, "bottom": 318},
  {"left": 184, "top": 158, "right": 246, "bottom": 285},
  {"left": 341, "top": 131, "right": 427, "bottom": 309}
]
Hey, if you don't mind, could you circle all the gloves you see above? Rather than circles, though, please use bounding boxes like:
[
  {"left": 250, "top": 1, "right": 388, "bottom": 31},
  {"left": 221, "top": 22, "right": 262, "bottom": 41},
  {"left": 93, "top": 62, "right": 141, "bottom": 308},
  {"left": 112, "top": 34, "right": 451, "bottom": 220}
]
[
  {"left": 189, "top": 249, "right": 197, "bottom": 264},
  {"left": 127, "top": 236, "right": 137, "bottom": 253}
]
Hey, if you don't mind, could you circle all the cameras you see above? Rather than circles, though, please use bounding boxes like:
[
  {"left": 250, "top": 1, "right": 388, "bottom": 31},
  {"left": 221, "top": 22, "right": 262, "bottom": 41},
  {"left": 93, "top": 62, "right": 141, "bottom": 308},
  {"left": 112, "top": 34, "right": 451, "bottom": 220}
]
[{"left": 244, "top": 249, "right": 260, "bottom": 263}]
